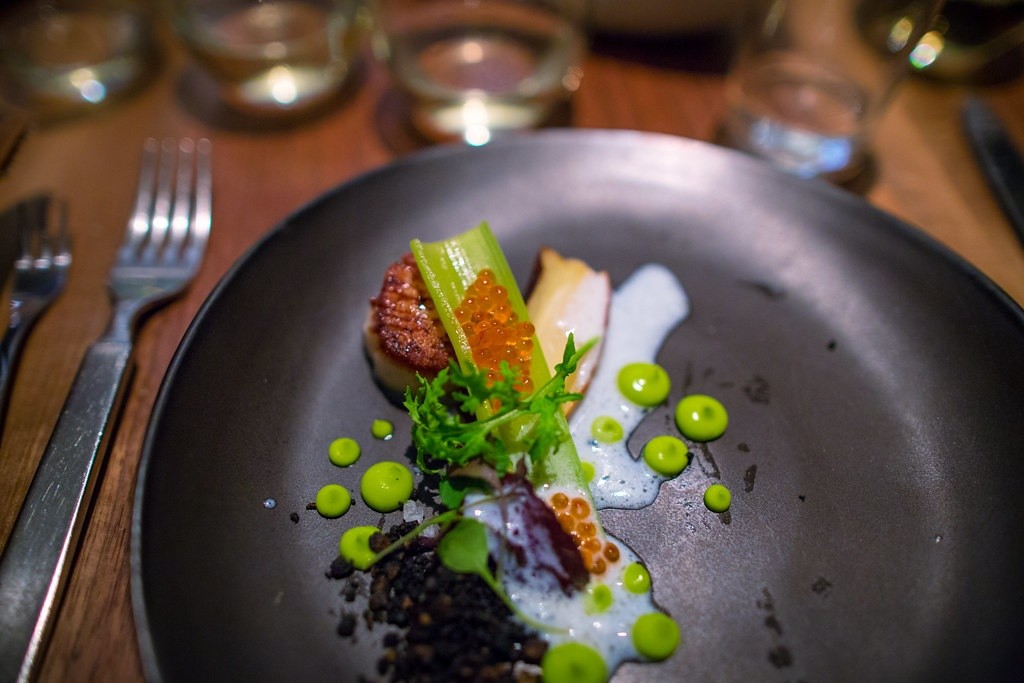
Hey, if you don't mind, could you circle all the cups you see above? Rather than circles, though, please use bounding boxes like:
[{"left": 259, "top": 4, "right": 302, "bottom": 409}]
[
  {"left": 721, "top": 1, "right": 942, "bottom": 184},
  {"left": 168, "top": 1, "right": 371, "bottom": 132},
  {"left": 379, "top": 1, "right": 583, "bottom": 145},
  {"left": 0, "top": 1, "right": 159, "bottom": 114}
]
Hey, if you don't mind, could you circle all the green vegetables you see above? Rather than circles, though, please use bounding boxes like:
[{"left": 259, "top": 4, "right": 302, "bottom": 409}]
[{"left": 355, "top": 333, "right": 603, "bottom": 636}]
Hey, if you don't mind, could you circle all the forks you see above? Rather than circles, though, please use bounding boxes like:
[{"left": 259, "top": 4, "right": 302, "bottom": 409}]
[
  {"left": 0, "top": 138, "right": 212, "bottom": 682},
  {"left": 0, "top": 193, "right": 68, "bottom": 442}
]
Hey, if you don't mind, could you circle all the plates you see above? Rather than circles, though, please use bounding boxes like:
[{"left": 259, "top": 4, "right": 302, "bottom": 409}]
[{"left": 132, "top": 129, "right": 1023, "bottom": 683}]
